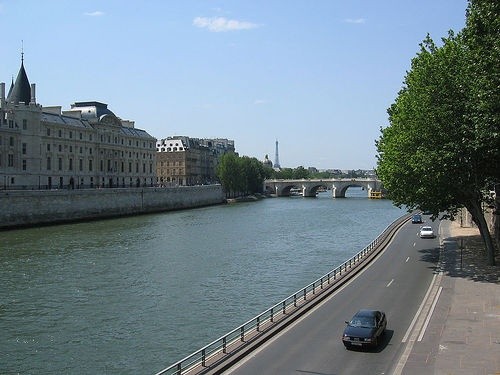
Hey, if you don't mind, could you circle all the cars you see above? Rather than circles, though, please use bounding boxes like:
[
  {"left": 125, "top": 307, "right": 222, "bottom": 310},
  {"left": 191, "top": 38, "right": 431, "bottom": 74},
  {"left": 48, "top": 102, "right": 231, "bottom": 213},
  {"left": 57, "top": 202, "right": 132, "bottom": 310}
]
[
  {"left": 342, "top": 309, "right": 386, "bottom": 350},
  {"left": 412, "top": 214, "right": 422, "bottom": 223},
  {"left": 419, "top": 225, "right": 434, "bottom": 237}
]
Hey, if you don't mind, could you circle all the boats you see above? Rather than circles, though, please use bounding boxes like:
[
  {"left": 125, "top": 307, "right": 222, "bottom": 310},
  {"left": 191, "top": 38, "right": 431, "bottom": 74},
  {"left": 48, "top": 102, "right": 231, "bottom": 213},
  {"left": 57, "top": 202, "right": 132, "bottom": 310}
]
[{"left": 369, "top": 191, "right": 382, "bottom": 199}]
